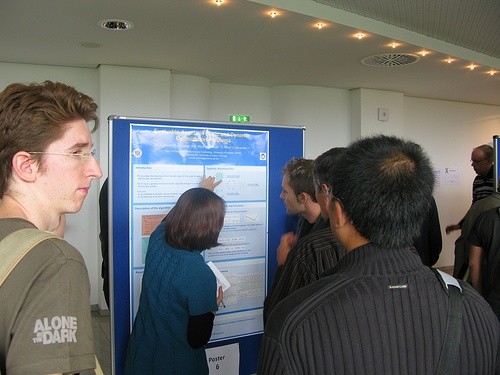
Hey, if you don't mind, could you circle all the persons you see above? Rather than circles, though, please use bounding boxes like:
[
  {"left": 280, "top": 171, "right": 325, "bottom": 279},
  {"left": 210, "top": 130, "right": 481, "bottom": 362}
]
[
  {"left": 0, "top": 80, "right": 103, "bottom": 375},
  {"left": 122, "top": 174, "right": 225, "bottom": 375},
  {"left": 256, "top": 135, "right": 500, "bottom": 375},
  {"left": 98, "top": 176, "right": 110, "bottom": 312}
]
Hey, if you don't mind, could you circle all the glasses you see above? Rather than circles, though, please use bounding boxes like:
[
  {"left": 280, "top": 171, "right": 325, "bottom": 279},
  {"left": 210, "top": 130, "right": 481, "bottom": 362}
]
[
  {"left": 470, "top": 158, "right": 488, "bottom": 164},
  {"left": 28, "top": 148, "right": 95, "bottom": 160}
]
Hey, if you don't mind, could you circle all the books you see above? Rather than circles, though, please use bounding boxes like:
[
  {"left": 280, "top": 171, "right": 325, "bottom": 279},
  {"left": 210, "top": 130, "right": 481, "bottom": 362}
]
[{"left": 206, "top": 261, "right": 231, "bottom": 298}]
